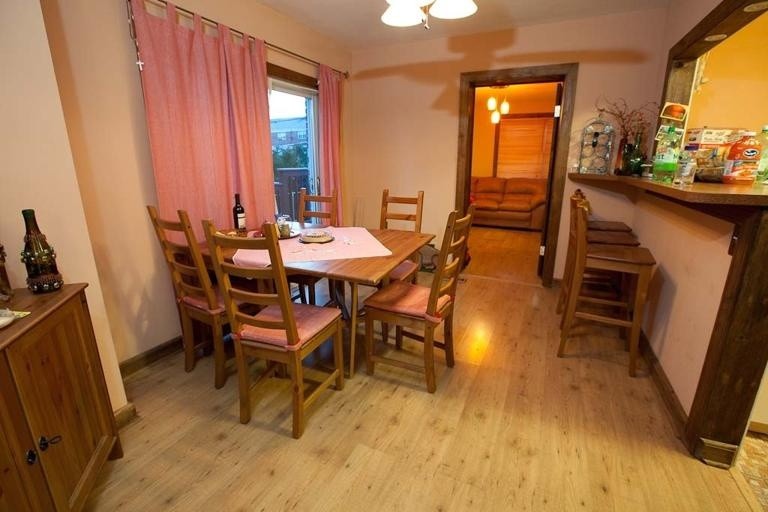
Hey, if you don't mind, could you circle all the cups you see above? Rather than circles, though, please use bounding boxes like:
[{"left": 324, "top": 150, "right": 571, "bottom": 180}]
[
  {"left": 674, "top": 160, "right": 698, "bottom": 186},
  {"left": 276, "top": 215, "right": 293, "bottom": 233}
]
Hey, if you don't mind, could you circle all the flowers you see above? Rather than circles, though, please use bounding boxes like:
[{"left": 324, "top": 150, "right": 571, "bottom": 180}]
[{"left": 592, "top": 92, "right": 665, "bottom": 141}]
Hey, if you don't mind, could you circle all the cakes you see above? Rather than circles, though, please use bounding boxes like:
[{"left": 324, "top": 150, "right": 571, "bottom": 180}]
[{"left": 301, "top": 231, "right": 333, "bottom": 243}]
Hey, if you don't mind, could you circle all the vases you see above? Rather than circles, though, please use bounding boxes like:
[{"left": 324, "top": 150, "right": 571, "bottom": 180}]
[{"left": 612, "top": 132, "right": 631, "bottom": 177}]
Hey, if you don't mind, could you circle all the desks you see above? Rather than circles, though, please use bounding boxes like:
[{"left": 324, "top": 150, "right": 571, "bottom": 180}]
[{"left": 198, "top": 222, "right": 437, "bottom": 378}]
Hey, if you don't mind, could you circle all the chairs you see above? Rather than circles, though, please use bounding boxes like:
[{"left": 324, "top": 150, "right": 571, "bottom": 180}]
[
  {"left": 284, "top": 188, "right": 426, "bottom": 311},
  {"left": 364, "top": 205, "right": 473, "bottom": 392},
  {"left": 551, "top": 188, "right": 658, "bottom": 381},
  {"left": 147, "top": 206, "right": 259, "bottom": 390},
  {"left": 200, "top": 219, "right": 344, "bottom": 439}
]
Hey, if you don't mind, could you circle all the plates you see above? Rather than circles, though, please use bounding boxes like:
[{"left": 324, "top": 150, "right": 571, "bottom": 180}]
[
  {"left": 299, "top": 236, "right": 334, "bottom": 243},
  {"left": 253, "top": 231, "right": 302, "bottom": 239}
]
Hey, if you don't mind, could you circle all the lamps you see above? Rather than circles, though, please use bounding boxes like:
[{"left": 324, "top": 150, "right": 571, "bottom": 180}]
[
  {"left": 484, "top": 83, "right": 511, "bottom": 126},
  {"left": 380, "top": 0, "right": 480, "bottom": 30}
]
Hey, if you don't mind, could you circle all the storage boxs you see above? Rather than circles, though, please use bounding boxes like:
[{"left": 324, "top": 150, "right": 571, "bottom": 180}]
[
  {"left": 687, "top": 142, "right": 730, "bottom": 166},
  {"left": 684, "top": 126, "right": 748, "bottom": 147}
]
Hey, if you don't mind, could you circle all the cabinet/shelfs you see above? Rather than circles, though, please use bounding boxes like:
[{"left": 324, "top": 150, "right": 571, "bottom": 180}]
[{"left": 0, "top": 282, "right": 127, "bottom": 512}]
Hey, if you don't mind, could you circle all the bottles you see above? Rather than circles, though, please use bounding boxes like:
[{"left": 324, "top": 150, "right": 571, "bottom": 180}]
[
  {"left": 624, "top": 132, "right": 644, "bottom": 177},
  {"left": 20, "top": 207, "right": 63, "bottom": 293},
  {"left": 652, "top": 125, "right": 678, "bottom": 183},
  {"left": 233, "top": 193, "right": 247, "bottom": 234},
  {"left": 723, "top": 124, "right": 768, "bottom": 186}
]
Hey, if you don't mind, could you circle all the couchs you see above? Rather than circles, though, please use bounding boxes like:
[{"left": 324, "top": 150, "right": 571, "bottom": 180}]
[{"left": 467, "top": 173, "right": 548, "bottom": 232}]
